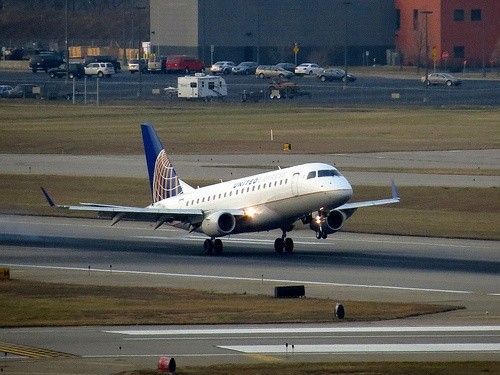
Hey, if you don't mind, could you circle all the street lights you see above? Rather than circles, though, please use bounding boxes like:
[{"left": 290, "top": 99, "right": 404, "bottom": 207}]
[
  {"left": 419, "top": 10, "right": 434, "bottom": 87},
  {"left": 341, "top": 1, "right": 351, "bottom": 88},
  {"left": 133, "top": 6, "right": 146, "bottom": 98}
]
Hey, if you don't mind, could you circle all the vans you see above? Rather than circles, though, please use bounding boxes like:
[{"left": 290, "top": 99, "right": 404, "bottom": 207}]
[
  {"left": 148, "top": 55, "right": 171, "bottom": 72},
  {"left": 81, "top": 57, "right": 121, "bottom": 72},
  {"left": 164, "top": 53, "right": 206, "bottom": 75}
]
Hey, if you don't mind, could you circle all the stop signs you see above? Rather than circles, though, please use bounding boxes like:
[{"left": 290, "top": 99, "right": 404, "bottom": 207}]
[{"left": 442, "top": 51, "right": 449, "bottom": 60}]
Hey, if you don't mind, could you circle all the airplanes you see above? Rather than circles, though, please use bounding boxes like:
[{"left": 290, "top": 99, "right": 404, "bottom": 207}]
[{"left": 38, "top": 122, "right": 403, "bottom": 256}]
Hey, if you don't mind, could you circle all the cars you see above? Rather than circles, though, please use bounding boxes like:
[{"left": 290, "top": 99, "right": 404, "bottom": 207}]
[
  {"left": 0, "top": 84, "right": 13, "bottom": 97},
  {"left": 295, "top": 63, "right": 325, "bottom": 77},
  {"left": 38, "top": 83, "right": 79, "bottom": 101},
  {"left": 83, "top": 62, "right": 116, "bottom": 78},
  {"left": 419, "top": 72, "right": 462, "bottom": 87},
  {"left": 210, "top": 60, "right": 238, "bottom": 74},
  {"left": 4, "top": 84, "right": 45, "bottom": 100},
  {"left": 316, "top": 68, "right": 357, "bottom": 84},
  {"left": 231, "top": 61, "right": 260, "bottom": 76},
  {"left": 0, "top": 45, "right": 25, "bottom": 60},
  {"left": 277, "top": 62, "right": 296, "bottom": 75},
  {"left": 127, "top": 58, "right": 148, "bottom": 74},
  {"left": 255, "top": 64, "right": 298, "bottom": 79}
]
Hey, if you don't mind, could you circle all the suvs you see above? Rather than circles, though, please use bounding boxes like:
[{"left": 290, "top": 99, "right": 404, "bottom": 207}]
[
  {"left": 47, "top": 61, "right": 85, "bottom": 78},
  {"left": 29, "top": 51, "right": 64, "bottom": 73}
]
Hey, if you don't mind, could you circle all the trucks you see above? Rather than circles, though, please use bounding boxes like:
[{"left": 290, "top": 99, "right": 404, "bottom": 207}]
[{"left": 177, "top": 72, "right": 226, "bottom": 102}]
